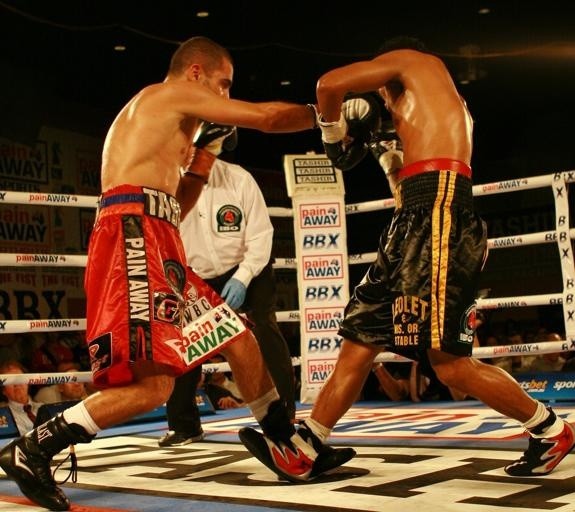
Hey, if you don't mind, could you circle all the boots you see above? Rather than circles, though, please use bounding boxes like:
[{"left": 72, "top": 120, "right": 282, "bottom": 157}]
[
  {"left": 0, "top": 410, "right": 98, "bottom": 512},
  {"left": 257, "top": 396, "right": 358, "bottom": 481}
]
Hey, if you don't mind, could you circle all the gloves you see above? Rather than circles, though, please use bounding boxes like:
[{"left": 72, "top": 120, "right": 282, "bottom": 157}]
[
  {"left": 220, "top": 277, "right": 247, "bottom": 312},
  {"left": 368, "top": 112, "right": 405, "bottom": 176},
  {"left": 180, "top": 117, "right": 239, "bottom": 186},
  {"left": 304, "top": 94, "right": 383, "bottom": 173}
]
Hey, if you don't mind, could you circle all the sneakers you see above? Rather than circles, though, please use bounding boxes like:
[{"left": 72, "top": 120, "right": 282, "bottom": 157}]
[
  {"left": 158, "top": 424, "right": 205, "bottom": 448},
  {"left": 504, "top": 420, "right": 575, "bottom": 477},
  {"left": 238, "top": 419, "right": 329, "bottom": 485}
]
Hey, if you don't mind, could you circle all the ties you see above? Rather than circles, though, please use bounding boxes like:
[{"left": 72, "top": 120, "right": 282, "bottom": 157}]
[{"left": 22, "top": 405, "right": 37, "bottom": 424}]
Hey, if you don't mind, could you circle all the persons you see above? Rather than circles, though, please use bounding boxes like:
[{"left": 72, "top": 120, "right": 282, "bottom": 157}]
[
  {"left": 154, "top": 147, "right": 297, "bottom": 451},
  {"left": 1, "top": 36, "right": 383, "bottom": 510},
  {"left": 359, "top": 325, "right": 575, "bottom": 403},
  {"left": 1, "top": 325, "right": 92, "bottom": 438},
  {"left": 236, "top": 32, "right": 575, "bottom": 482},
  {"left": 195, "top": 354, "right": 249, "bottom": 410}
]
